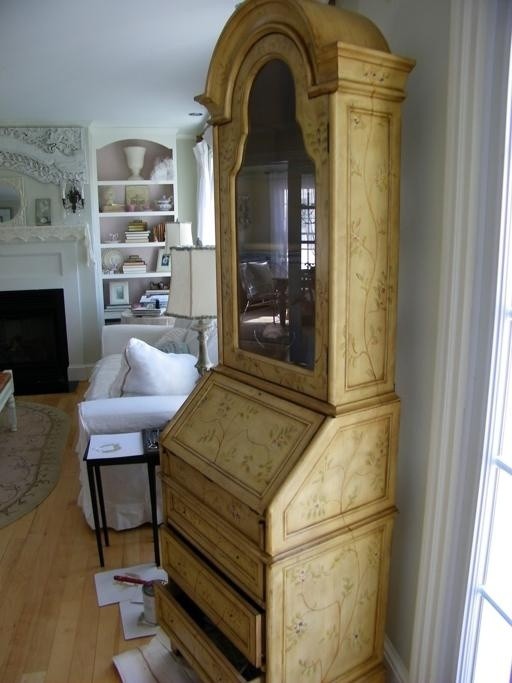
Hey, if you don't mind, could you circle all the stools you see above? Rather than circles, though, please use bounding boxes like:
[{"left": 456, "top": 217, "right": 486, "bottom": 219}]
[{"left": 83, "top": 432, "right": 162, "bottom": 568}]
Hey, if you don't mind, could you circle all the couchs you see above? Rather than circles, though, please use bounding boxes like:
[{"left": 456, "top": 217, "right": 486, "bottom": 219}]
[{"left": 73, "top": 317, "right": 220, "bottom": 532}]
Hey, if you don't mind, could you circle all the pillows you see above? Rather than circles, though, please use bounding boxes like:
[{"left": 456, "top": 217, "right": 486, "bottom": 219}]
[{"left": 122, "top": 338, "right": 199, "bottom": 396}]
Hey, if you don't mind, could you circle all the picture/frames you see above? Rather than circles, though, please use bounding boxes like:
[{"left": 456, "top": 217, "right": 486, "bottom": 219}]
[
  {"left": 125, "top": 185, "right": 152, "bottom": 212},
  {"left": 108, "top": 281, "right": 131, "bottom": 306},
  {"left": 156, "top": 248, "right": 172, "bottom": 274}
]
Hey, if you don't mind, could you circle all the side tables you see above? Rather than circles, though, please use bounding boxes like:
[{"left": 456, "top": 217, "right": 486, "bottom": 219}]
[{"left": 120, "top": 308, "right": 176, "bottom": 326}]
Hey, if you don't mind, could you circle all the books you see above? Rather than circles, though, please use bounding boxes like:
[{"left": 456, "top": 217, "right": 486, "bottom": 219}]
[
  {"left": 120, "top": 254, "right": 148, "bottom": 274},
  {"left": 132, "top": 307, "right": 165, "bottom": 315},
  {"left": 104, "top": 304, "right": 132, "bottom": 312},
  {"left": 124, "top": 230, "right": 151, "bottom": 243}
]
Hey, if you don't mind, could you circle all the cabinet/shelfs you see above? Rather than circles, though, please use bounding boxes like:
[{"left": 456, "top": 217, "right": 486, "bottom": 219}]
[
  {"left": 90, "top": 125, "right": 178, "bottom": 349},
  {"left": 154, "top": 0, "right": 417, "bottom": 682}
]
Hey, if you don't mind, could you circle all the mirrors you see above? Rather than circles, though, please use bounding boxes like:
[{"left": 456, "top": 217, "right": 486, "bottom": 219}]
[{"left": 0, "top": 176, "right": 25, "bottom": 227}]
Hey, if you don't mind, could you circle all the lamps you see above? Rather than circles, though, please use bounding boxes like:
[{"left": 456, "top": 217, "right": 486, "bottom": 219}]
[
  {"left": 165, "top": 219, "right": 194, "bottom": 257},
  {"left": 166, "top": 246, "right": 217, "bottom": 378},
  {"left": 59, "top": 178, "right": 86, "bottom": 214}
]
